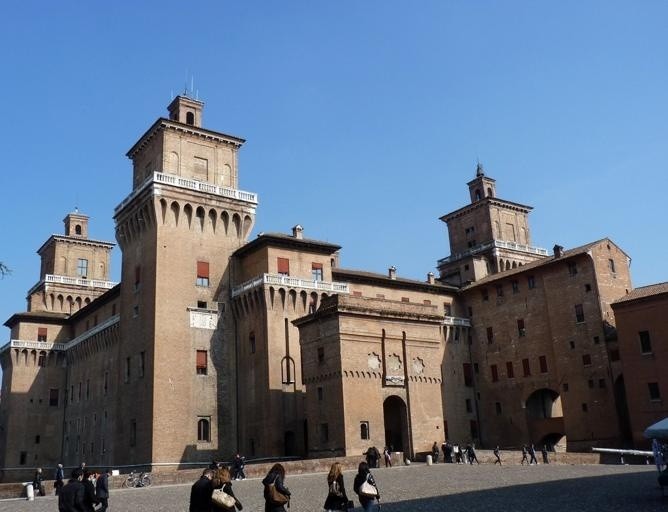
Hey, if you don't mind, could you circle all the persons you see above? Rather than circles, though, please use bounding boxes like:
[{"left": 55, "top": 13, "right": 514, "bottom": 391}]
[
  {"left": 541, "top": 444, "right": 549, "bottom": 464},
  {"left": 432, "top": 441, "right": 480, "bottom": 465},
  {"left": 324, "top": 462, "right": 348, "bottom": 512},
  {"left": 363, "top": 446, "right": 392, "bottom": 468},
  {"left": 529, "top": 444, "right": 540, "bottom": 465},
  {"left": 354, "top": 462, "right": 380, "bottom": 512},
  {"left": 189, "top": 453, "right": 247, "bottom": 512},
  {"left": 521, "top": 444, "right": 529, "bottom": 466},
  {"left": 262, "top": 464, "right": 291, "bottom": 512},
  {"left": 54, "top": 462, "right": 112, "bottom": 512},
  {"left": 32, "top": 467, "right": 45, "bottom": 496},
  {"left": 494, "top": 445, "right": 502, "bottom": 466}
]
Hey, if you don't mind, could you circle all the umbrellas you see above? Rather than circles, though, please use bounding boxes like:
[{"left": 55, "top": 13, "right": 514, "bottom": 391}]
[{"left": 644, "top": 417, "right": 668, "bottom": 440}]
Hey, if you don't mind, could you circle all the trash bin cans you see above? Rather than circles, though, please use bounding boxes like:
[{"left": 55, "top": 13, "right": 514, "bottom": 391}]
[{"left": 426, "top": 455, "right": 433, "bottom": 466}]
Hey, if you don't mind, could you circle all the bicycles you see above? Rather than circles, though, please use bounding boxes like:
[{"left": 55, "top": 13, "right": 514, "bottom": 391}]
[{"left": 124, "top": 469, "right": 153, "bottom": 488}]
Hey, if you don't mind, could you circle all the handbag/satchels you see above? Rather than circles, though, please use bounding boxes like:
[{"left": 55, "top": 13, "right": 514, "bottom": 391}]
[
  {"left": 212, "top": 489, "right": 236, "bottom": 508},
  {"left": 359, "top": 481, "right": 377, "bottom": 497},
  {"left": 270, "top": 483, "right": 289, "bottom": 504},
  {"left": 329, "top": 481, "right": 343, "bottom": 496}
]
